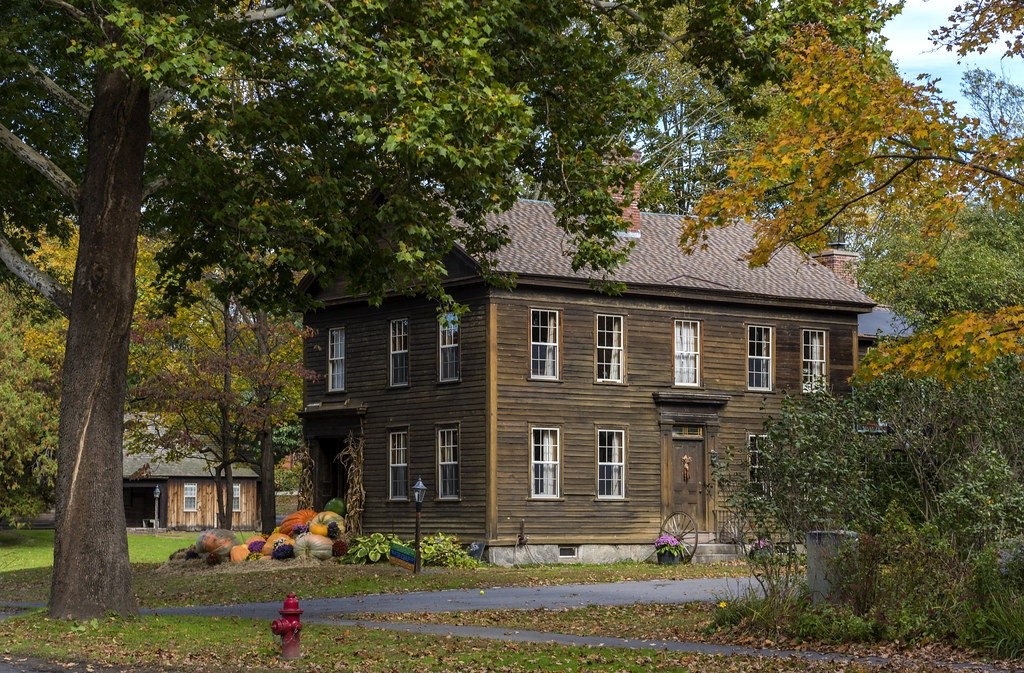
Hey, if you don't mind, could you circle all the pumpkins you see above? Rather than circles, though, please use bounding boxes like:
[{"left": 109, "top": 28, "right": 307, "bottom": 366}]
[{"left": 196, "top": 498, "right": 347, "bottom": 560}]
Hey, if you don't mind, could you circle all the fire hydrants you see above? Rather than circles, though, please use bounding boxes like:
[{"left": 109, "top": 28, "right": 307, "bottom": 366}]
[{"left": 270, "top": 590, "right": 307, "bottom": 662}]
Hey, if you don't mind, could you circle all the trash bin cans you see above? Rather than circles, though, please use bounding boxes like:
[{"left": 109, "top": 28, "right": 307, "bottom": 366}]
[{"left": 806, "top": 530, "right": 859, "bottom": 605}]
